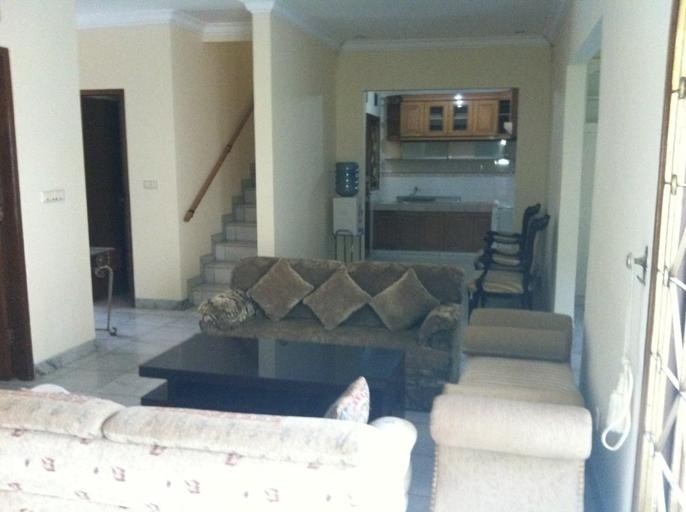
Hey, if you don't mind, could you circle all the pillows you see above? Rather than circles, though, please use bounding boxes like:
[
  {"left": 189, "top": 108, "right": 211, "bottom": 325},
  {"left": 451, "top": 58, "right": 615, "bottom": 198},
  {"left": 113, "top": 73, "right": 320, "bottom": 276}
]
[
  {"left": 367, "top": 267, "right": 439, "bottom": 333},
  {"left": 302, "top": 264, "right": 369, "bottom": 333},
  {"left": 461, "top": 325, "right": 568, "bottom": 361},
  {"left": 442, "top": 381, "right": 569, "bottom": 402},
  {"left": 245, "top": 257, "right": 315, "bottom": 324},
  {"left": 324, "top": 375, "right": 370, "bottom": 424}
]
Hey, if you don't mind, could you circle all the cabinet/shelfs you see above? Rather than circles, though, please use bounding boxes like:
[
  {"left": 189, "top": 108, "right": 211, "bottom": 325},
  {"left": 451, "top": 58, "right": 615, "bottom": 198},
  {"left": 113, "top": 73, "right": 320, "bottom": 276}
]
[
  {"left": 373, "top": 210, "right": 491, "bottom": 255},
  {"left": 398, "top": 92, "right": 503, "bottom": 139}
]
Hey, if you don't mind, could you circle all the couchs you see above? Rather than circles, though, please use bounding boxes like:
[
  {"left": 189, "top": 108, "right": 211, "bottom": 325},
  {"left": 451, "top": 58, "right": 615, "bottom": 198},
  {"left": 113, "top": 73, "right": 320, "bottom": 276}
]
[
  {"left": 197, "top": 256, "right": 466, "bottom": 413},
  {"left": 0, "top": 386, "right": 418, "bottom": 511}
]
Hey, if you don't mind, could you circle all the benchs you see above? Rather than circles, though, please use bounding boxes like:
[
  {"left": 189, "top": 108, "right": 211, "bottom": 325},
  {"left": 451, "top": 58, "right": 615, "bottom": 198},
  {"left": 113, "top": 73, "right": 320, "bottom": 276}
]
[{"left": 428, "top": 309, "right": 595, "bottom": 512}]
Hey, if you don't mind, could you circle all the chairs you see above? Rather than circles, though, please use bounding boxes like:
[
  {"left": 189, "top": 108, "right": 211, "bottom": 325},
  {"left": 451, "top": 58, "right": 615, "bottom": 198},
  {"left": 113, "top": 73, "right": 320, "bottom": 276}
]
[{"left": 467, "top": 202, "right": 551, "bottom": 312}]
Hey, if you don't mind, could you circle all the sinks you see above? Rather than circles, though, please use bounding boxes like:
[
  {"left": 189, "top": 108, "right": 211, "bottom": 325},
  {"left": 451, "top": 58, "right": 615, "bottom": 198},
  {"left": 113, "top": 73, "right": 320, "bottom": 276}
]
[
  {"left": 432, "top": 196, "right": 460, "bottom": 204},
  {"left": 396, "top": 195, "right": 434, "bottom": 202}
]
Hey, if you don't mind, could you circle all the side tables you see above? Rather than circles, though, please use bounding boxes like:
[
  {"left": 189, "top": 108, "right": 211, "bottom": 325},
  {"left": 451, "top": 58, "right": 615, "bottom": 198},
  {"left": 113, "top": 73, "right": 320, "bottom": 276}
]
[{"left": 332, "top": 229, "right": 362, "bottom": 262}]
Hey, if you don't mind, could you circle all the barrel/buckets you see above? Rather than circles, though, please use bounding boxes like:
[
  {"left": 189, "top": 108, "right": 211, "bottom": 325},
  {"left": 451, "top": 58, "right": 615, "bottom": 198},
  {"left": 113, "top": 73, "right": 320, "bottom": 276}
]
[{"left": 334, "top": 161, "right": 361, "bottom": 197}]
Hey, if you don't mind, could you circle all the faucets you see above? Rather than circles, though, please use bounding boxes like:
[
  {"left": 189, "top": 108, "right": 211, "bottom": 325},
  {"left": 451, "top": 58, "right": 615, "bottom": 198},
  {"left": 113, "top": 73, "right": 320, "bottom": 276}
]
[{"left": 409, "top": 186, "right": 419, "bottom": 195}]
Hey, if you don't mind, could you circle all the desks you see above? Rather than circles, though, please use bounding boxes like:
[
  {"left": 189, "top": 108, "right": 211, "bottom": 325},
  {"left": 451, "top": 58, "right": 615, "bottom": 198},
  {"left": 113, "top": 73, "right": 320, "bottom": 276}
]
[{"left": 90, "top": 246, "right": 118, "bottom": 336}]
